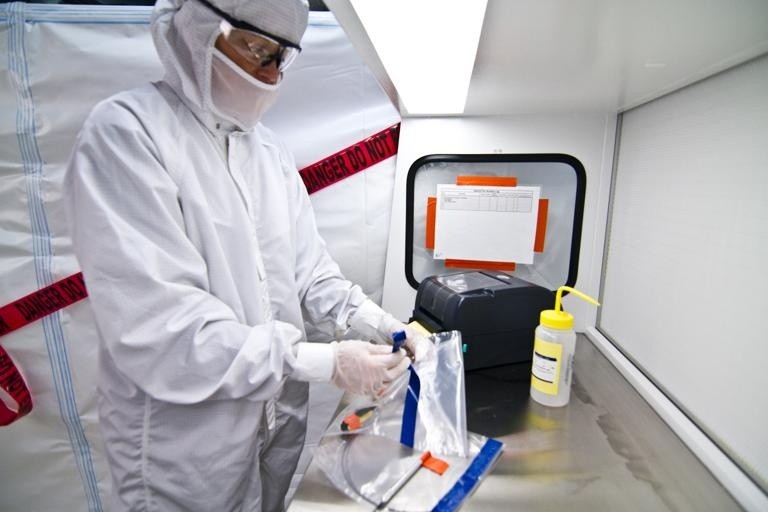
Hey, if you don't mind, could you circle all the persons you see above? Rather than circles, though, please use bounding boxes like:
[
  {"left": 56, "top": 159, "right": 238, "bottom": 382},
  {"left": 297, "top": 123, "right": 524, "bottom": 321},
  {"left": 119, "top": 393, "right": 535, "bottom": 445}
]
[{"left": 63, "top": 1, "right": 442, "bottom": 512}]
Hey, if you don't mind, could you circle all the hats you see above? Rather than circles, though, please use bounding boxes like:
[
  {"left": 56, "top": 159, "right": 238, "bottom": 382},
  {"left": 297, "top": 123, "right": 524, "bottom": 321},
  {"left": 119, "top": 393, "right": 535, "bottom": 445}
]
[{"left": 152, "top": 0, "right": 310, "bottom": 134}]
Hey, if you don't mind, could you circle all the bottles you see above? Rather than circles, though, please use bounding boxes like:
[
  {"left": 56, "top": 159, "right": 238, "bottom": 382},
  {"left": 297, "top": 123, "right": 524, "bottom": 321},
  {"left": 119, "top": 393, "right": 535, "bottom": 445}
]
[
  {"left": 528, "top": 310, "right": 577, "bottom": 408},
  {"left": 525, "top": 403, "right": 568, "bottom": 487}
]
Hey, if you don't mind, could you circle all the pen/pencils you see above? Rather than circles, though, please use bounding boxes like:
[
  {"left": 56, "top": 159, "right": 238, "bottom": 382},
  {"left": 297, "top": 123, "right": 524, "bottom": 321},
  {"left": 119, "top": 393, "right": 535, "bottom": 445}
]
[{"left": 373, "top": 452, "right": 430, "bottom": 512}]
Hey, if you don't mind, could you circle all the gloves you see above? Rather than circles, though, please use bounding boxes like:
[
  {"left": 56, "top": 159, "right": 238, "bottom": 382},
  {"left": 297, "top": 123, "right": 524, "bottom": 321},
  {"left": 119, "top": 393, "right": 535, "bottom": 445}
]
[
  {"left": 329, "top": 338, "right": 412, "bottom": 401},
  {"left": 370, "top": 314, "right": 436, "bottom": 375}
]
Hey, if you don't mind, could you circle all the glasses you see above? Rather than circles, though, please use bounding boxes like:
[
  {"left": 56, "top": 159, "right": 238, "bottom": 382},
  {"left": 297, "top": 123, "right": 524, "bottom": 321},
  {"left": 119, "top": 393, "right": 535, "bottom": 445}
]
[{"left": 215, "top": 18, "right": 303, "bottom": 74}]
